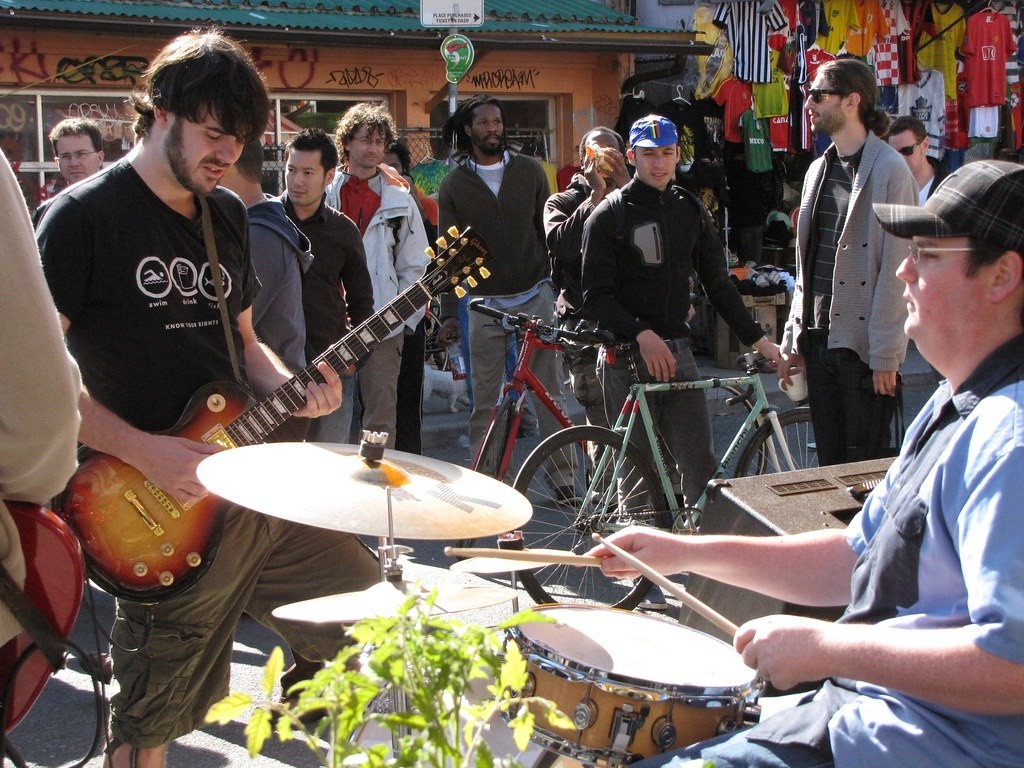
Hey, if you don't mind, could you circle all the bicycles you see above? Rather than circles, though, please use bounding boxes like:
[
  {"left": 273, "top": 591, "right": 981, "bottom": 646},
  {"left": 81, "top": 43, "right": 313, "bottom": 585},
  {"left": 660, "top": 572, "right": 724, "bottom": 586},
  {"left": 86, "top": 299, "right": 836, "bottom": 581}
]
[
  {"left": 452, "top": 295, "right": 610, "bottom": 565},
  {"left": 499, "top": 325, "right": 820, "bottom": 624}
]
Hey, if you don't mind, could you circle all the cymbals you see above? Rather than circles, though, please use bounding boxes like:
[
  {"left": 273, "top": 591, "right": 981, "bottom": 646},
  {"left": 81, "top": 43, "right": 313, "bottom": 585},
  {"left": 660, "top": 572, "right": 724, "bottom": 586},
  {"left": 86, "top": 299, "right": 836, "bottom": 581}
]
[
  {"left": 194, "top": 440, "right": 536, "bottom": 542},
  {"left": 448, "top": 548, "right": 579, "bottom": 574},
  {"left": 271, "top": 581, "right": 522, "bottom": 626}
]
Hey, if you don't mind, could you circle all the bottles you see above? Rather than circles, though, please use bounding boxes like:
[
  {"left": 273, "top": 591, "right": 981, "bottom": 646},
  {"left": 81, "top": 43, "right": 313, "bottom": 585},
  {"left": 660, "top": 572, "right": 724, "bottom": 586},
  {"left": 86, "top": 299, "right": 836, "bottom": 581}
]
[{"left": 448, "top": 336, "right": 467, "bottom": 380}]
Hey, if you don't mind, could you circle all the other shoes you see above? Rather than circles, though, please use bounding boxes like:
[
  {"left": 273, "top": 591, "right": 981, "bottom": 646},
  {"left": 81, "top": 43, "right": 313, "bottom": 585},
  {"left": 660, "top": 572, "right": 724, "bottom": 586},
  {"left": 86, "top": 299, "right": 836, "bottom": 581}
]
[
  {"left": 518, "top": 413, "right": 538, "bottom": 430},
  {"left": 554, "top": 485, "right": 581, "bottom": 504},
  {"left": 80, "top": 648, "right": 113, "bottom": 684},
  {"left": 637, "top": 583, "right": 667, "bottom": 609},
  {"left": 591, "top": 492, "right": 619, "bottom": 505}
]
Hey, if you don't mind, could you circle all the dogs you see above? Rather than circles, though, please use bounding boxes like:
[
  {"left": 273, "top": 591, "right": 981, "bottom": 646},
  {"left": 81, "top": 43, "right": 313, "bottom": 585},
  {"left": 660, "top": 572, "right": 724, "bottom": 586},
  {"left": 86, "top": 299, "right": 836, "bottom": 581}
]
[{"left": 421, "top": 365, "right": 470, "bottom": 413}]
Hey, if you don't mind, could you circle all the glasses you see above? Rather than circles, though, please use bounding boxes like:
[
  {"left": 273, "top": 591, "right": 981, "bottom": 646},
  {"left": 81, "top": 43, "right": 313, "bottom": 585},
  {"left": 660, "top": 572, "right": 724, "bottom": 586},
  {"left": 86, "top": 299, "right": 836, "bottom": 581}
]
[
  {"left": 908, "top": 241, "right": 974, "bottom": 264},
  {"left": 353, "top": 136, "right": 389, "bottom": 148},
  {"left": 897, "top": 135, "right": 926, "bottom": 156},
  {"left": 808, "top": 88, "right": 840, "bottom": 104},
  {"left": 55, "top": 150, "right": 97, "bottom": 163}
]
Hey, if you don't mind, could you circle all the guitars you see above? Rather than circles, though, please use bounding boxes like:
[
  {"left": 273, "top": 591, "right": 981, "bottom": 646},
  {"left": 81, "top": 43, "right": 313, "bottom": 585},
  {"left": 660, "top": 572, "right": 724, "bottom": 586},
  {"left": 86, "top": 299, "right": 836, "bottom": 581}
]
[
  {"left": 54, "top": 221, "right": 494, "bottom": 603},
  {"left": 1, "top": 496, "right": 88, "bottom": 736}
]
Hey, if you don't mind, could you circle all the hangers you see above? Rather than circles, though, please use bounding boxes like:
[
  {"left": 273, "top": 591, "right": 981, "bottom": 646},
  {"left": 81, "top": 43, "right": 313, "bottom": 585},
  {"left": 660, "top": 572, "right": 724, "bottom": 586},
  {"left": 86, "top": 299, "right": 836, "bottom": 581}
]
[
  {"left": 980, "top": 0, "right": 997, "bottom": 13},
  {"left": 672, "top": 84, "right": 691, "bottom": 105},
  {"left": 419, "top": 144, "right": 436, "bottom": 163},
  {"left": 807, "top": 39, "right": 821, "bottom": 52},
  {"left": 750, "top": 95, "right": 755, "bottom": 111},
  {"left": 530, "top": 143, "right": 539, "bottom": 157},
  {"left": 633, "top": 81, "right": 646, "bottom": 100}
]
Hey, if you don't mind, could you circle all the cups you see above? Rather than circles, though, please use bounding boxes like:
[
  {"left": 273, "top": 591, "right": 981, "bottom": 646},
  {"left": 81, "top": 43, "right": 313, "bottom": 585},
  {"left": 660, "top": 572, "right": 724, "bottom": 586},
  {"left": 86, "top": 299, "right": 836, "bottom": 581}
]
[
  {"left": 596, "top": 147, "right": 615, "bottom": 178},
  {"left": 778, "top": 367, "right": 808, "bottom": 401}
]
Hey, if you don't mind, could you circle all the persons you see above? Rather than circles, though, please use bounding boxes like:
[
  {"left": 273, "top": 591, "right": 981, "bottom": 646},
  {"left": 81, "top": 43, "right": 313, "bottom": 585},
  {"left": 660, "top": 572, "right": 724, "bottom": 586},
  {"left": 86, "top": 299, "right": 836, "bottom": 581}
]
[
  {"left": 777, "top": 60, "right": 918, "bottom": 468},
  {"left": 572, "top": 158, "right": 1024, "bottom": 768},
  {"left": 34, "top": 22, "right": 381, "bottom": 768},
  {"left": 1, "top": 145, "right": 86, "bottom": 647},
  {"left": 277, "top": 126, "right": 377, "bottom": 443},
  {"left": 327, "top": 97, "right": 431, "bottom": 449},
  {"left": 542, "top": 125, "right": 689, "bottom": 521},
  {"left": 582, "top": 114, "right": 780, "bottom": 530},
  {"left": 889, "top": 116, "right": 952, "bottom": 207},
  {"left": 382, "top": 141, "right": 437, "bottom": 456},
  {"left": 431, "top": 93, "right": 589, "bottom": 501},
  {"left": 215, "top": 135, "right": 314, "bottom": 443},
  {"left": 49, "top": 118, "right": 105, "bottom": 186}
]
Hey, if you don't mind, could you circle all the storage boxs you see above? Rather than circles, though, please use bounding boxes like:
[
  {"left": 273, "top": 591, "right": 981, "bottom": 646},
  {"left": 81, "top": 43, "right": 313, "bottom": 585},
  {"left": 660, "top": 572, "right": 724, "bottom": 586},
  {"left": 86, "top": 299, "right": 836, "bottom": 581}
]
[{"left": 715, "top": 306, "right": 776, "bottom": 370}]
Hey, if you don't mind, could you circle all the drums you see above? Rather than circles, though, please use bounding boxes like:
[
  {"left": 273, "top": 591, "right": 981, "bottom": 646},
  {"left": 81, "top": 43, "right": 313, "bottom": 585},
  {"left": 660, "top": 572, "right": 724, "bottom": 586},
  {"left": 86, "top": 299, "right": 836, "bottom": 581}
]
[
  {"left": 503, "top": 602, "right": 765, "bottom": 765},
  {"left": 532, "top": 743, "right": 617, "bottom": 768}
]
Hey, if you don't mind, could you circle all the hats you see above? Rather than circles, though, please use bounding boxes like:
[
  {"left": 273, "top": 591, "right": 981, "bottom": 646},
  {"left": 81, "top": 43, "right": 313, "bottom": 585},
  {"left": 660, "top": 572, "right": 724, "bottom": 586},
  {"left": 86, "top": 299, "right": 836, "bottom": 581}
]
[
  {"left": 872, "top": 160, "right": 1024, "bottom": 256},
  {"left": 628, "top": 115, "right": 678, "bottom": 151}
]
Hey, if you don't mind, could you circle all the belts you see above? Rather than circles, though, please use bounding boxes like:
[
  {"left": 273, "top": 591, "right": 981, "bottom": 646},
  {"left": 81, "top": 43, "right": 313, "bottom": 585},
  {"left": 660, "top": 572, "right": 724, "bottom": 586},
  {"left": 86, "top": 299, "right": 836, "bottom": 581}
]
[{"left": 663, "top": 337, "right": 691, "bottom": 352}]
